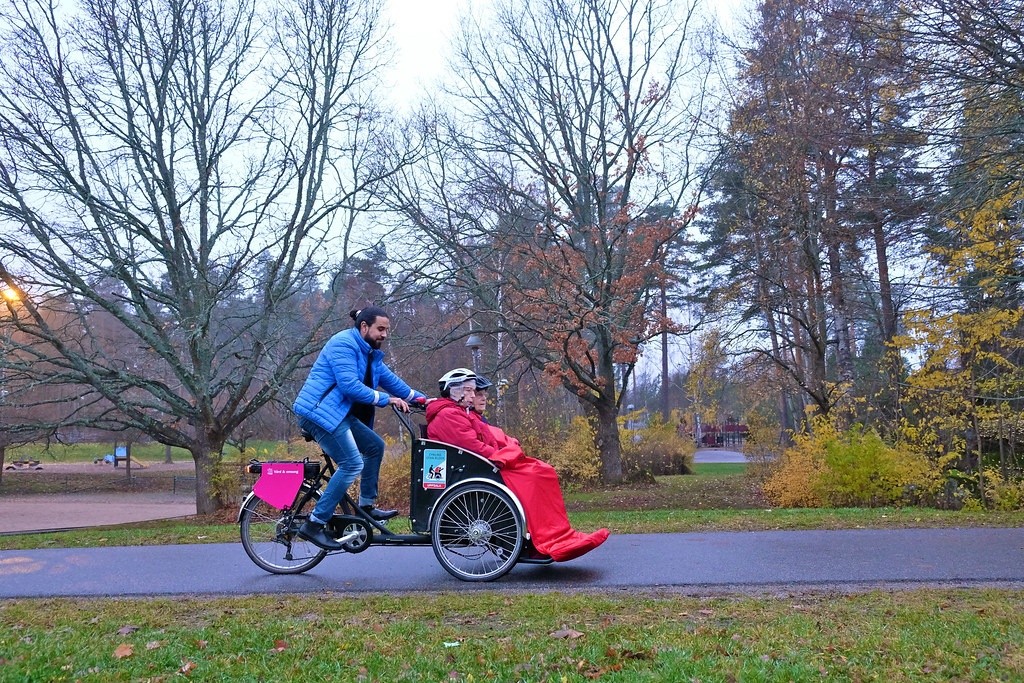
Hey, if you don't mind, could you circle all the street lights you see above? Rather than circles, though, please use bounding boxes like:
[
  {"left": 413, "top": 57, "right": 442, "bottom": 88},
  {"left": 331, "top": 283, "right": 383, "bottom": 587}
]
[{"left": 466, "top": 335, "right": 484, "bottom": 374}]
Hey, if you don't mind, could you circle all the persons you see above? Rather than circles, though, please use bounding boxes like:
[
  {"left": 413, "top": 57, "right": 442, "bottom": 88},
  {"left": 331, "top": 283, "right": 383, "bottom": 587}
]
[
  {"left": 293, "top": 306, "right": 438, "bottom": 550},
  {"left": 474, "top": 375, "right": 494, "bottom": 426},
  {"left": 425, "top": 368, "right": 611, "bottom": 563}
]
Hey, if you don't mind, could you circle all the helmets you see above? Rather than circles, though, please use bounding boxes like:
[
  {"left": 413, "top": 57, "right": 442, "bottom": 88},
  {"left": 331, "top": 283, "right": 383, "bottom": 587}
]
[
  {"left": 475, "top": 376, "right": 494, "bottom": 390},
  {"left": 438, "top": 367, "right": 477, "bottom": 391}
]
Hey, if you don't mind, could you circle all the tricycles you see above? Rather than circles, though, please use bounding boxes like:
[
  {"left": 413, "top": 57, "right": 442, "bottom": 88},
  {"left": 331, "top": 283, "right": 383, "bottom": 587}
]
[{"left": 236, "top": 403, "right": 553, "bottom": 583}]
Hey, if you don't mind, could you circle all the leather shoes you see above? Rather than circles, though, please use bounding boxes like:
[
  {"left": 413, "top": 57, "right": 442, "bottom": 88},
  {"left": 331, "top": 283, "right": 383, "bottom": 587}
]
[
  {"left": 355, "top": 507, "right": 398, "bottom": 521},
  {"left": 297, "top": 522, "right": 342, "bottom": 550}
]
